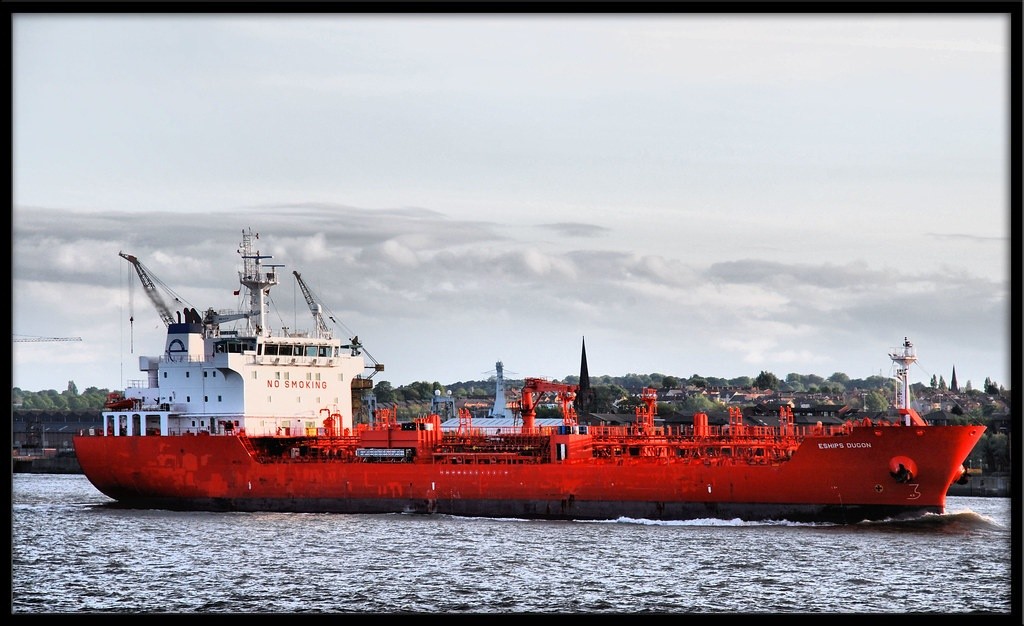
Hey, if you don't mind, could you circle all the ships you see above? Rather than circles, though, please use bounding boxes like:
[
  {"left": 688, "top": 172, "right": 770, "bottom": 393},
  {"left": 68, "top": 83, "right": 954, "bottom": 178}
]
[{"left": 75, "top": 228, "right": 988, "bottom": 520}]
